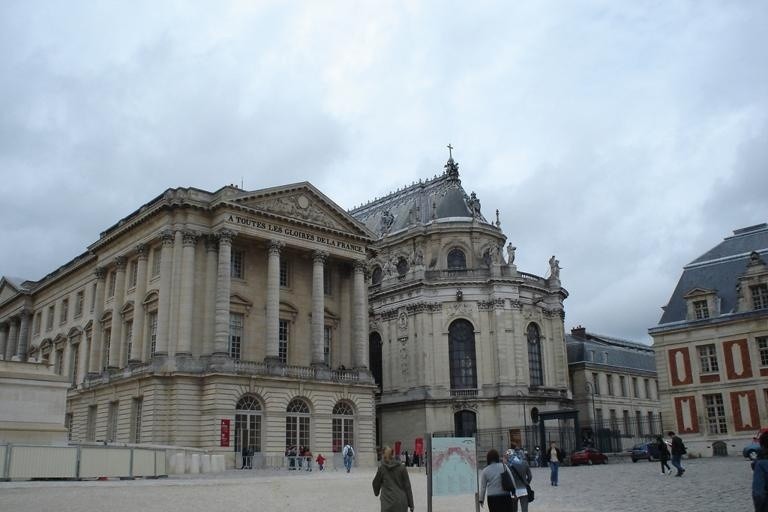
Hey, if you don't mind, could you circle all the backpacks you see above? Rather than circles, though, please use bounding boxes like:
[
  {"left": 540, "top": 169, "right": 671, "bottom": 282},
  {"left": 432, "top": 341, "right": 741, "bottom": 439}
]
[{"left": 345, "top": 446, "right": 353, "bottom": 456}]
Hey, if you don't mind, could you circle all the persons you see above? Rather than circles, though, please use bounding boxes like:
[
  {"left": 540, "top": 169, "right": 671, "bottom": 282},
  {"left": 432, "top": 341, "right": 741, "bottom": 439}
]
[
  {"left": 372, "top": 446, "right": 416, "bottom": 512},
  {"left": 478, "top": 449, "right": 515, "bottom": 511},
  {"left": 513, "top": 444, "right": 544, "bottom": 466},
  {"left": 751, "top": 431, "right": 768, "bottom": 511},
  {"left": 507, "top": 242, "right": 517, "bottom": 264},
  {"left": 446, "top": 158, "right": 461, "bottom": 179},
  {"left": 401, "top": 447, "right": 428, "bottom": 469},
  {"left": 367, "top": 242, "right": 425, "bottom": 284},
  {"left": 548, "top": 440, "right": 562, "bottom": 484},
  {"left": 343, "top": 440, "right": 352, "bottom": 470},
  {"left": 555, "top": 259, "right": 564, "bottom": 278},
  {"left": 381, "top": 208, "right": 392, "bottom": 232},
  {"left": 656, "top": 435, "right": 672, "bottom": 475},
  {"left": 283, "top": 443, "right": 326, "bottom": 472},
  {"left": 503, "top": 449, "right": 532, "bottom": 510},
  {"left": 240, "top": 443, "right": 255, "bottom": 470},
  {"left": 668, "top": 430, "right": 688, "bottom": 476},
  {"left": 470, "top": 191, "right": 481, "bottom": 217},
  {"left": 549, "top": 255, "right": 557, "bottom": 278},
  {"left": 490, "top": 239, "right": 501, "bottom": 263}
]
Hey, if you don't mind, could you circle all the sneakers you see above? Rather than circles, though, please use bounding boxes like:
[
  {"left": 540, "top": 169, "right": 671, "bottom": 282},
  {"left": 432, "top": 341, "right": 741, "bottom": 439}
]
[
  {"left": 675, "top": 469, "right": 685, "bottom": 476},
  {"left": 551, "top": 481, "right": 558, "bottom": 486},
  {"left": 661, "top": 469, "right": 672, "bottom": 474},
  {"left": 346, "top": 468, "right": 350, "bottom": 473}
]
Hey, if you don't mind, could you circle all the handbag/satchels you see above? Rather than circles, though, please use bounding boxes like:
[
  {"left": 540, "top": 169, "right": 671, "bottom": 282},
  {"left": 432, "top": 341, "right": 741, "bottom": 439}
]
[
  {"left": 500, "top": 471, "right": 514, "bottom": 492},
  {"left": 526, "top": 485, "right": 534, "bottom": 502}
]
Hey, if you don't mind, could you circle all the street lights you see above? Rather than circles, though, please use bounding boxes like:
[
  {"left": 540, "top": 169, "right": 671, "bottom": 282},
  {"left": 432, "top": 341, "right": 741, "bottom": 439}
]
[
  {"left": 584, "top": 381, "right": 599, "bottom": 451},
  {"left": 514, "top": 389, "right": 531, "bottom": 468}
]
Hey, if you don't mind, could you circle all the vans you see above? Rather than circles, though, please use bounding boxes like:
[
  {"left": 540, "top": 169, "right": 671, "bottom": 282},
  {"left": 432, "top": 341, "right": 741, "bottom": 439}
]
[{"left": 630, "top": 441, "right": 671, "bottom": 462}]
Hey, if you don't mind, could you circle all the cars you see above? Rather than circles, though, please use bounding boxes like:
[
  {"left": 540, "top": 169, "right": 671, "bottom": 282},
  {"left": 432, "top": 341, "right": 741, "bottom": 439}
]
[
  {"left": 571, "top": 448, "right": 609, "bottom": 465},
  {"left": 742, "top": 428, "right": 768, "bottom": 461}
]
